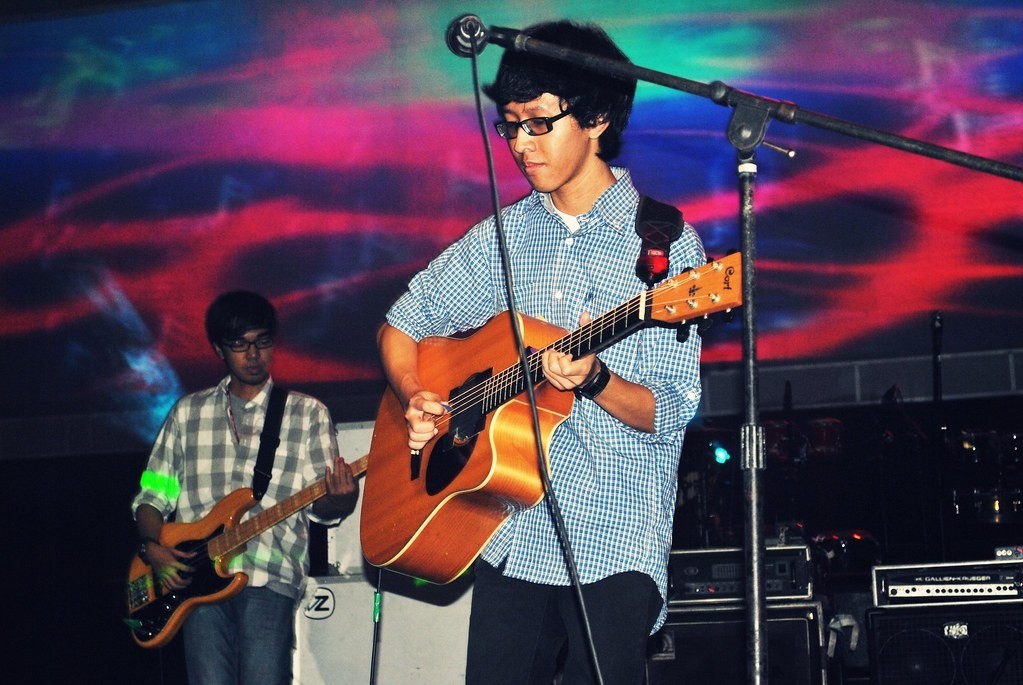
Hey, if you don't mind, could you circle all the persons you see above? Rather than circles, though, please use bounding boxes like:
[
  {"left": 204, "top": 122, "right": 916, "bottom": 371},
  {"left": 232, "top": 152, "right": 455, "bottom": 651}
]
[
  {"left": 377, "top": 19, "right": 708, "bottom": 685},
  {"left": 131, "top": 291, "right": 356, "bottom": 685}
]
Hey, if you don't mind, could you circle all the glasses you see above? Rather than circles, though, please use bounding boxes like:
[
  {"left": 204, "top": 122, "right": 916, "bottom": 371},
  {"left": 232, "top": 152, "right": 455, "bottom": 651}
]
[
  {"left": 495, "top": 109, "right": 572, "bottom": 139},
  {"left": 222, "top": 337, "right": 273, "bottom": 352}
]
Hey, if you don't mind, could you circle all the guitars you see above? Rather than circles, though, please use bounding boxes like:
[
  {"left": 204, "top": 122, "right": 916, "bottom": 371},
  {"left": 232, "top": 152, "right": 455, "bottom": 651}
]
[
  {"left": 127, "top": 452, "right": 372, "bottom": 651},
  {"left": 359, "top": 244, "right": 747, "bottom": 586}
]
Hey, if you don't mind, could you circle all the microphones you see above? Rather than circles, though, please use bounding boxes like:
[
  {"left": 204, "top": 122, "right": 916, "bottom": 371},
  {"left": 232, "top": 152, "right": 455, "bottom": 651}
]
[{"left": 445, "top": 13, "right": 488, "bottom": 58}]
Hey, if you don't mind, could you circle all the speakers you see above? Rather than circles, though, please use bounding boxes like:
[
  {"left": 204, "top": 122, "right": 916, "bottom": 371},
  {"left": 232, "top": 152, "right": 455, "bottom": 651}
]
[
  {"left": 868, "top": 602, "right": 1023, "bottom": 685},
  {"left": 646, "top": 600, "right": 828, "bottom": 685}
]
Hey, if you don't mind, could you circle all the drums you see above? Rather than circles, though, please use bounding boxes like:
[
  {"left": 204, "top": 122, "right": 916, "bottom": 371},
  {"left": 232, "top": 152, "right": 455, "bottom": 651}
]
[
  {"left": 809, "top": 528, "right": 882, "bottom": 587},
  {"left": 963, "top": 485, "right": 1023, "bottom": 525},
  {"left": 761, "top": 418, "right": 802, "bottom": 472},
  {"left": 804, "top": 416, "right": 846, "bottom": 464},
  {"left": 956, "top": 429, "right": 1023, "bottom": 469}
]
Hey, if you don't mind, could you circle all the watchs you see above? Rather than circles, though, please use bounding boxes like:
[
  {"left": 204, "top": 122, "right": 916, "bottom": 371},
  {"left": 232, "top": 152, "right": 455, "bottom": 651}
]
[
  {"left": 574, "top": 357, "right": 611, "bottom": 402},
  {"left": 138, "top": 537, "right": 159, "bottom": 559}
]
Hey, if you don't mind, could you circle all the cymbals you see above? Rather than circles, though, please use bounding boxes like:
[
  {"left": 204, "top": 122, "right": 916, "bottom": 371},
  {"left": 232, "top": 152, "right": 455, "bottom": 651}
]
[{"left": 684, "top": 426, "right": 736, "bottom": 437}]
